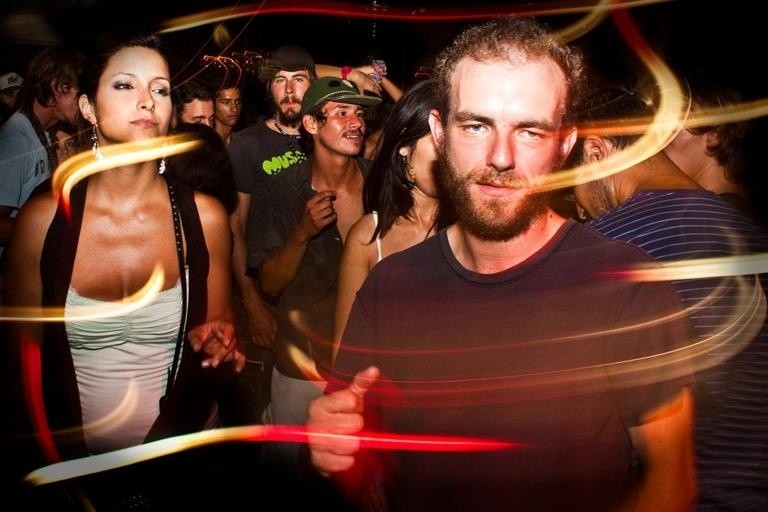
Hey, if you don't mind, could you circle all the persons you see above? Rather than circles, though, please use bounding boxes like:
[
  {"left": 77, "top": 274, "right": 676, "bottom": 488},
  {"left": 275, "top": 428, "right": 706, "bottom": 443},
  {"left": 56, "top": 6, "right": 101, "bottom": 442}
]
[
  {"left": 240, "top": 77, "right": 377, "bottom": 474},
  {"left": 0, "top": 40, "right": 768, "bottom": 363},
  {"left": 306, "top": 17, "right": 701, "bottom": 511},
  {"left": 559, "top": 51, "right": 768, "bottom": 512},
  {"left": 1, "top": 30, "right": 246, "bottom": 511}
]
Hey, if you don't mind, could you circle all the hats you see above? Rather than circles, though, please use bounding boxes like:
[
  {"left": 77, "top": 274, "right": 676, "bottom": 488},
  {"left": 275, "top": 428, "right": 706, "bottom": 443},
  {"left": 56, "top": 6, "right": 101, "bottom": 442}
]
[
  {"left": 267, "top": 47, "right": 317, "bottom": 89},
  {"left": 300, "top": 75, "right": 382, "bottom": 116}
]
